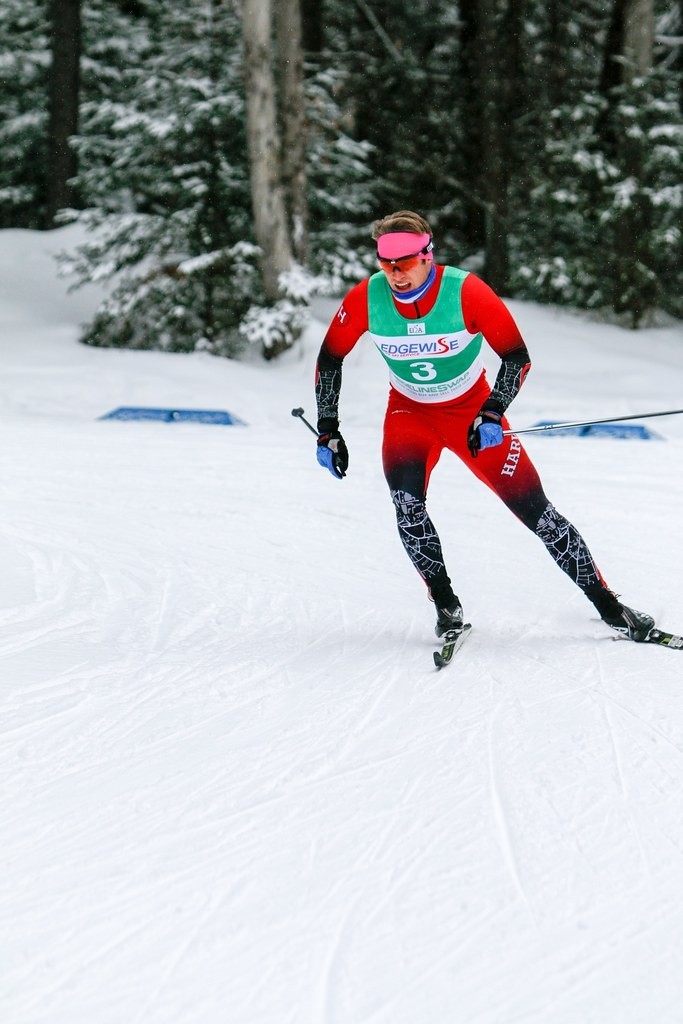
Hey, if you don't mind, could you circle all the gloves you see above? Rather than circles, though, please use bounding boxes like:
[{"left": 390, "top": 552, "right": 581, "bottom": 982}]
[
  {"left": 467, "top": 399, "right": 504, "bottom": 450},
  {"left": 316, "top": 416, "right": 349, "bottom": 479}
]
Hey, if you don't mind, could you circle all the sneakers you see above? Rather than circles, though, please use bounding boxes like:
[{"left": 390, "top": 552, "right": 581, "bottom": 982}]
[
  {"left": 424, "top": 574, "right": 464, "bottom": 639},
  {"left": 584, "top": 585, "right": 655, "bottom": 643}
]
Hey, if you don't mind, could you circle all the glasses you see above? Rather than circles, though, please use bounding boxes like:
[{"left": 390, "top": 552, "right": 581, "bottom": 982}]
[{"left": 376, "top": 238, "right": 434, "bottom": 274}]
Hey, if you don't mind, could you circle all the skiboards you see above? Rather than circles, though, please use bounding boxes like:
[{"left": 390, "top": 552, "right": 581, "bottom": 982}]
[{"left": 431, "top": 622, "right": 683, "bottom": 667}]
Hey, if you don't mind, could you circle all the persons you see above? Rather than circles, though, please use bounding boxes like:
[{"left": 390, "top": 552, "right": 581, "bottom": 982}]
[{"left": 313, "top": 209, "right": 655, "bottom": 641}]
